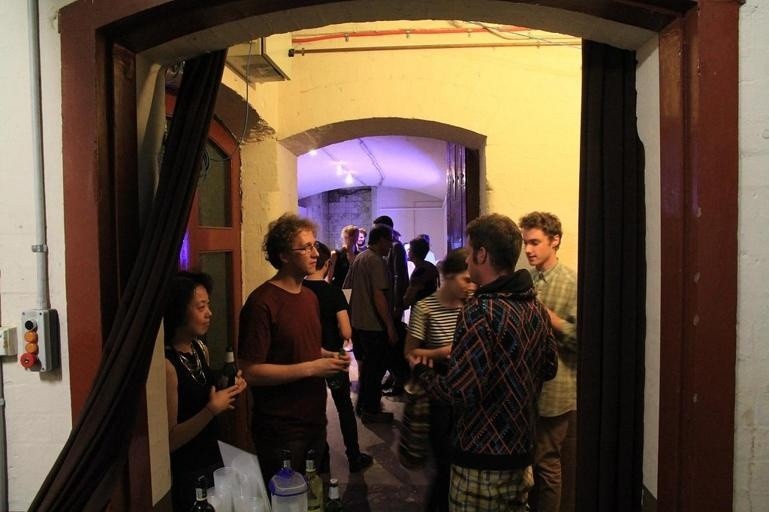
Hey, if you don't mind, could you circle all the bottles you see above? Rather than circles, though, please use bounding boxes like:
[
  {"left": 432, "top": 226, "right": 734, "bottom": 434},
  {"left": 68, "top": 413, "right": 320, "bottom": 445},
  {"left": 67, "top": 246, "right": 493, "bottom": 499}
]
[
  {"left": 327, "top": 350, "right": 354, "bottom": 396},
  {"left": 221, "top": 347, "right": 241, "bottom": 405},
  {"left": 189, "top": 476, "right": 212, "bottom": 512},
  {"left": 304, "top": 449, "right": 324, "bottom": 512},
  {"left": 323, "top": 479, "right": 349, "bottom": 512},
  {"left": 270, "top": 447, "right": 309, "bottom": 512}
]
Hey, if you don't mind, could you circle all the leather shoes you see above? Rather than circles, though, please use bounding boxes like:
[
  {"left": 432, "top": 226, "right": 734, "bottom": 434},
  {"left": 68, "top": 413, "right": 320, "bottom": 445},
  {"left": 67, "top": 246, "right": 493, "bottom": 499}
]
[{"left": 381, "top": 380, "right": 405, "bottom": 396}]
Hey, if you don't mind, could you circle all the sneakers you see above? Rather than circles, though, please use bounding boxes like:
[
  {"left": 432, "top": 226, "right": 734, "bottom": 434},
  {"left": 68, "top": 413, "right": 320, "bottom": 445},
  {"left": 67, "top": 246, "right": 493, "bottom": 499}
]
[
  {"left": 355, "top": 406, "right": 394, "bottom": 424},
  {"left": 349, "top": 453, "right": 373, "bottom": 473}
]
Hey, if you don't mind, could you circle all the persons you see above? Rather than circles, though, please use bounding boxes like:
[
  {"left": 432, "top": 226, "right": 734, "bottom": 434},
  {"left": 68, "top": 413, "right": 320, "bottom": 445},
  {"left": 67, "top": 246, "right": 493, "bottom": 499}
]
[
  {"left": 301, "top": 241, "right": 374, "bottom": 484},
  {"left": 407, "top": 212, "right": 560, "bottom": 512},
  {"left": 519, "top": 210, "right": 578, "bottom": 512},
  {"left": 238, "top": 212, "right": 352, "bottom": 505},
  {"left": 397, "top": 245, "right": 479, "bottom": 511},
  {"left": 327, "top": 214, "right": 440, "bottom": 425},
  {"left": 164, "top": 269, "right": 248, "bottom": 511}
]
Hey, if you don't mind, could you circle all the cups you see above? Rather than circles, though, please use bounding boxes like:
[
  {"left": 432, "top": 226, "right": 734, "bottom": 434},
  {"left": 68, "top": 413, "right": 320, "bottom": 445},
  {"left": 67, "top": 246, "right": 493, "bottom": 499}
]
[{"left": 207, "top": 454, "right": 265, "bottom": 512}]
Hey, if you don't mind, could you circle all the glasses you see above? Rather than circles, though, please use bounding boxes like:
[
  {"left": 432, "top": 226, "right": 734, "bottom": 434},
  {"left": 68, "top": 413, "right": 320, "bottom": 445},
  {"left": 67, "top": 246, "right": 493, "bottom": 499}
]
[{"left": 290, "top": 243, "right": 319, "bottom": 253}]
